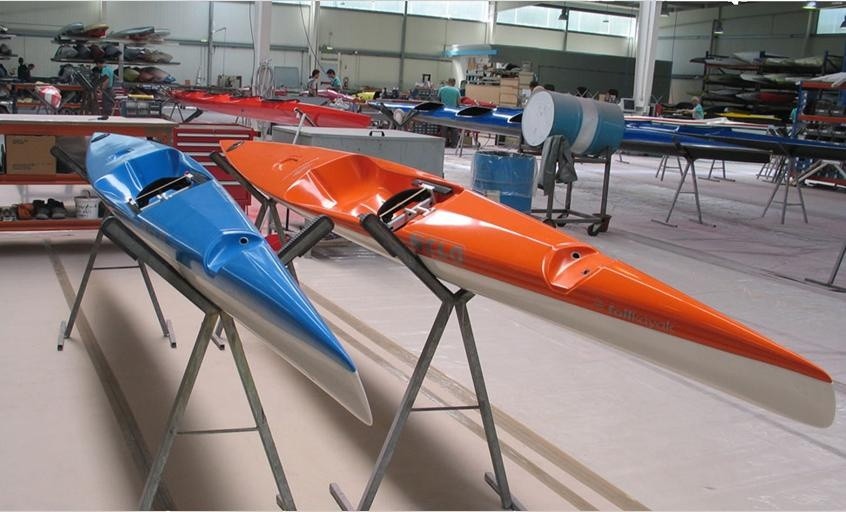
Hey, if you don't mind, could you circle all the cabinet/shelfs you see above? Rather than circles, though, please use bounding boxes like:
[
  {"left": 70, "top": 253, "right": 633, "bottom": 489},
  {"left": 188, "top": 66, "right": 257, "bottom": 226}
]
[
  {"left": 465, "top": 76, "right": 518, "bottom": 108},
  {"left": 683, "top": 49, "right": 824, "bottom": 122},
  {"left": 0, "top": 33, "right": 182, "bottom": 115}
]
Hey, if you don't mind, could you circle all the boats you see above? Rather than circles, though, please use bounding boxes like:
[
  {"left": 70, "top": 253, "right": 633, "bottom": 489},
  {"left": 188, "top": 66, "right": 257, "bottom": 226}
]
[
  {"left": 623, "top": 117, "right": 840, "bottom": 135},
  {"left": 85, "top": 132, "right": 374, "bottom": 428},
  {"left": 162, "top": 88, "right": 372, "bottom": 128},
  {"left": 366, "top": 100, "right": 846, "bottom": 169},
  {"left": 685, "top": 49, "right": 846, "bottom": 116},
  {"left": 219, "top": 138, "right": 837, "bottom": 428},
  {"left": 0, "top": 24, "right": 175, "bottom": 110}
]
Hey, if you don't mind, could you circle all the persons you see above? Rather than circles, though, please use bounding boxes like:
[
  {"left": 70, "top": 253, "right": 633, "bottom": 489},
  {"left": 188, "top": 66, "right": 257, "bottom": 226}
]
[
  {"left": 437, "top": 79, "right": 461, "bottom": 148},
  {"left": 683, "top": 96, "right": 704, "bottom": 119},
  {"left": 307, "top": 69, "right": 320, "bottom": 96},
  {"left": 789, "top": 100, "right": 798, "bottom": 124},
  {"left": 96, "top": 56, "right": 114, "bottom": 115},
  {"left": 18, "top": 57, "right": 35, "bottom": 82},
  {"left": 460, "top": 80, "right": 467, "bottom": 96},
  {"left": 326, "top": 69, "right": 342, "bottom": 92}
]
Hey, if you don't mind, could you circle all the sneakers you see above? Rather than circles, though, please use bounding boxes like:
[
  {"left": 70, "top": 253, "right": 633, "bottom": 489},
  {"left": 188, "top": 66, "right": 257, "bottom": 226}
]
[
  {"left": 52, "top": 207, "right": 68, "bottom": 219},
  {"left": 34, "top": 205, "right": 50, "bottom": 220}
]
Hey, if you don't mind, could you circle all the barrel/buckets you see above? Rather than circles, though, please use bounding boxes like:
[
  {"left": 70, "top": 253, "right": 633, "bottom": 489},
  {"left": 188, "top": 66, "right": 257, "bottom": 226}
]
[
  {"left": 471, "top": 150, "right": 534, "bottom": 216},
  {"left": 75, "top": 190, "right": 101, "bottom": 219},
  {"left": 522, "top": 90, "right": 625, "bottom": 156}
]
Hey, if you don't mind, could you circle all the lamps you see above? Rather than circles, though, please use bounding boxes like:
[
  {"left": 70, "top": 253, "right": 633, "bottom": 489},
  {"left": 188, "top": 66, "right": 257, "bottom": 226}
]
[
  {"left": 714, "top": 20, "right": 724, "bottom": 34},
  {"left": 558, "top": 8, "right": 568, "bottom": 20},
  {"left": 660, "top": 3, "right": 669, "bottom": 17},
  {"left": 802, "top": 1, "right": 818, "bottom": 9}
]
